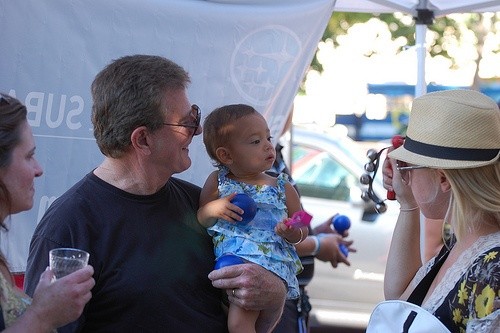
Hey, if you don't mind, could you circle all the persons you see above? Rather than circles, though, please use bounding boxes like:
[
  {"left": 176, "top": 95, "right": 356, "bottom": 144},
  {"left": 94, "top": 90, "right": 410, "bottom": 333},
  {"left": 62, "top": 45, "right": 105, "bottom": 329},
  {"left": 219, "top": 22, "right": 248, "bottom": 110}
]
[
  {"left": 424, "top": 217, "right": 444, "bottom": 263},
  {"left": 265, "top": 105, "right": 357, "bottom": 333},
  {"left": 382, "top": 89, "right": 500, "bottom": 333},
  {"left": 24, "top": 54, "right": 286, "bottom": 333},
  {"left": 0, "top": 93, "right": 95, "bottom": 333},
  {"left": 198, "top": 103, "right": 309, "bottom": 333}
]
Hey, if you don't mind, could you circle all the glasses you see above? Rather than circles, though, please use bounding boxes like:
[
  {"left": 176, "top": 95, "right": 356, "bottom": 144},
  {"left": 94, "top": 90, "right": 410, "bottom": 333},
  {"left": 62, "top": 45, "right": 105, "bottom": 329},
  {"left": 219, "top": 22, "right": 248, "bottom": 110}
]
[
  {"left": 138, "top": 104, "right": 201, "bottom": 137},
  {"left": 396, "top": 160, "right": 427, "bottom": 181},
  {"left": 0, "top": 93, "right": 12, "bottom": 104}
]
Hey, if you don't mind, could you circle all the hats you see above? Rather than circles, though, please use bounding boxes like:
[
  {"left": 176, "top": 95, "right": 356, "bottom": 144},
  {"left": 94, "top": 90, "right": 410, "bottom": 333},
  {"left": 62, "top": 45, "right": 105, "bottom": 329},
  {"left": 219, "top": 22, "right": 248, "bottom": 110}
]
[{"left": 387, "top": 90, "right": 500, "bottom": 170}]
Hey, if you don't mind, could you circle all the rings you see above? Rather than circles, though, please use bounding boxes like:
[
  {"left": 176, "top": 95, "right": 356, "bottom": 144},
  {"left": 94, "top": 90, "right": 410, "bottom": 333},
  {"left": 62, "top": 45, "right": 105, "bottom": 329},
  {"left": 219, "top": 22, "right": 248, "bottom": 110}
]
[{"left": 232, "top": 290, "right": 235, "bottom": 297}]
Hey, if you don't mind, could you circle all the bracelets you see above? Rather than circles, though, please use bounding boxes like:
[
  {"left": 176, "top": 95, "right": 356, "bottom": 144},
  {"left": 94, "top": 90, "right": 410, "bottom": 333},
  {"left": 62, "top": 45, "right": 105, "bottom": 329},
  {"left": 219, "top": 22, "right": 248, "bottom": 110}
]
[
  {"left": 311, "top": 235, "right": 320, "bottom": 255},
  {"left": 400, "top": 207, "right": 419, "bottom": 210},
  {"left": 284, "top": 227, "right": 303, "bottom": 244}
]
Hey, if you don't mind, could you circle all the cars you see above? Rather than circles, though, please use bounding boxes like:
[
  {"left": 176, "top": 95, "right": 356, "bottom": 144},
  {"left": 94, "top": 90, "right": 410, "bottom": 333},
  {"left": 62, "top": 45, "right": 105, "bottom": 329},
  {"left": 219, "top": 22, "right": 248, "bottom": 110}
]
[{"left": 290, "top": 123, "right": 425, "bottom": 330}]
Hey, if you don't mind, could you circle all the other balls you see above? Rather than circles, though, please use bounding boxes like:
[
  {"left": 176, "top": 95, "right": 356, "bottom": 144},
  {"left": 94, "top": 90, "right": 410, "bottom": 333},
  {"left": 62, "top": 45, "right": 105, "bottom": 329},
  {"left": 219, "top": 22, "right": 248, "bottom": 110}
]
[
  {"left": 230, "top": 194, "right": 258, "bottom": 225},
  {"left": 333, "top": 215, "right": 352, "bottom": 231},
  {"left": 338, "top": 244, "right": 348, "bottom": 262}
]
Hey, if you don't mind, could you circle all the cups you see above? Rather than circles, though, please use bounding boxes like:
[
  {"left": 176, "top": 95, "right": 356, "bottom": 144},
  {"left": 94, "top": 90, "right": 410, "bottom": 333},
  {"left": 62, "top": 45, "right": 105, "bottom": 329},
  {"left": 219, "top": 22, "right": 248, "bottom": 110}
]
[{"left": 49, "top": 246, "right": 90, "bottom": 281}]
